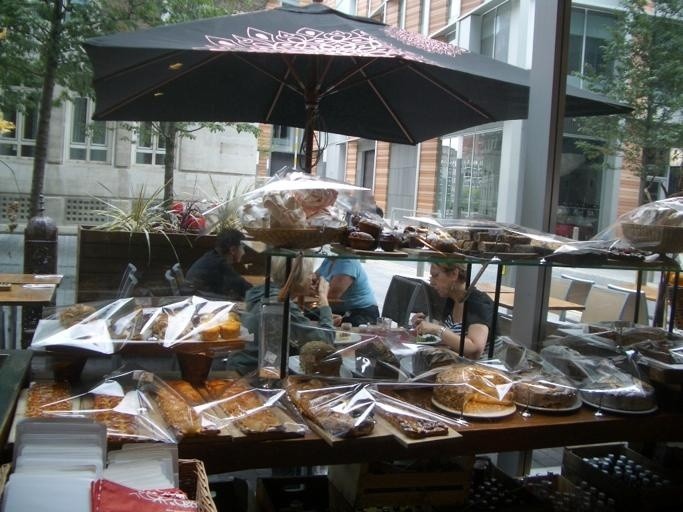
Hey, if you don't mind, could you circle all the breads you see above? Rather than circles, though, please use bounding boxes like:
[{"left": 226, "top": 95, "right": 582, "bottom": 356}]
[
  {"left": 23, "top": 378, "right": 71, "bottom": 420},
  {"left": 205, "top": 376, "right": 286, "bottom": 435},
  {"left": 274, "top": 371, "right": 373, "bottom": 437},
  {"left": 374, "top": 388, "right": 447, "bottom": 443},
  {"left": 93, "top": 384, "right": 138, "bottom": 439},
  {"left": 150, "top": 378, "right": 221, "bottom": 437}
]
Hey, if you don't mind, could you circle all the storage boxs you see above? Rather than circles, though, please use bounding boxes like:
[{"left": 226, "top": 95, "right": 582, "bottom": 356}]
[
  {"left": 327, "top": 480, "right": 353, "bottom": 511},
  {"left": 327, "top": 454, "right": 475, "bottom": 509},
  {"left": 465, "top": 457, "right": 550, "bottom": 512},
  {"left": 520, "top": 475, "right": 617, "bottom": 512},
  {"left": 562, "top": 442, "right": 678, "bottom": 511},
  {"left": 256, "top": 475, "right": 327, "bottom": 512}
]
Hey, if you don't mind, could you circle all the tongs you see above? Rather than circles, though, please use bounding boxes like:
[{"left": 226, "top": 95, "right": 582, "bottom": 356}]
[
  {"left": 275, "top": 395, "right": 311, "bottom": 434},
  {"left": 277, "top": 254, "right": 303, "bottom": 302}
]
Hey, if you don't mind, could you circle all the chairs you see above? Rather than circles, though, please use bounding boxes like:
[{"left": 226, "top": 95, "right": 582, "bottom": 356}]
[
  {"left": 164, "top": 269, "right": 183, "bottom": 371},
  {"left": 113, "top": 262, "right": 138, "bottom": 297},
  {"left": 382, "top": 275, "right": 422, "bottom": 329},
  {"left": 118, "top": 274, "right": 138, "bottom": 299},
  {"left": 172, "top": 262, "right": 193, "bottom": 296}
]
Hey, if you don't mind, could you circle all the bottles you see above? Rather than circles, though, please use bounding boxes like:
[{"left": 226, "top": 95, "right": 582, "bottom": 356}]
[{"left": 470, "top": 451, "right": 670, "bottom": 511}]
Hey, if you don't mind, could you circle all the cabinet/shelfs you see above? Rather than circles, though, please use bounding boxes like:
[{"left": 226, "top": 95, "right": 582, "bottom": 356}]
[{"left": 1, "top": 238, "right": 683, "bottom": 512}]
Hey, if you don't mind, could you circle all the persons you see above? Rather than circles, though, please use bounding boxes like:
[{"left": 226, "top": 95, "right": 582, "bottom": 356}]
[
  {"left": 171, "top": 203, "right": 199, "bottom": 228},
  {"left": 244, "top": 257, "right": 337, "bottom": 354},
  {"left": 411, "top": 260, "right": 503, "bottom": 360},
  {"left": 191, "top": 228, "right": 252, "bottom": 298},
  {"left": 313, "top": 258, "right": 380, "bottom": 325}
]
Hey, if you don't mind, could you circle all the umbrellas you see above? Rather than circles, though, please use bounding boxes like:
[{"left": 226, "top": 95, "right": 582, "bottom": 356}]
[{"left": 77, "top": 2, "right": 636, "bottom": 174}]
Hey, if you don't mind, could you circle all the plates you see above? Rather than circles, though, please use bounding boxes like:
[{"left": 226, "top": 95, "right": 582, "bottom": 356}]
[
  {"left": 581, "top": 400, "right": 658, "bottom": 414},
  {"left": 343, "top": 353, "right": 408, "bottom": 382},
  {"left": 416, "top": 333, "right": 442, "bottom": 346},
  {"left": 399, "top": 355, "right": 435, "bottom": 383},
  {"left": 334, "top": 330, "right": 361, "bottom": 345},
  {"left": 288, "top": 355, "right": 354, "bottom": 379},
  {"left": 515, "top": 394, "right": 582, "bottom": 414},
  {"left": 431, "top": 395, "right": 515, "bottom": 421}
]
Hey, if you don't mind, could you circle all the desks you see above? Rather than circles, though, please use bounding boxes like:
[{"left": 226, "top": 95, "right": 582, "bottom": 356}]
[
  {"left": 241, "top": 274, "right": 266, "bottom": 289},
  {"left": 0, "top": 274, "right": 64, "bottom": 287},
  {"left": 0, "top": 284, "right": 56, "bottom": 348}
]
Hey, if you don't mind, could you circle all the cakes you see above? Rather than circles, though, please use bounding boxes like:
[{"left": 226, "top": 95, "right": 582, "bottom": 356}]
[
  {"left": 354, "top": 341, "right": 398, "bottom": 380},
  {"left": 445, "top": 224, "right": 545, "bottom": 252},
  {"left": 297, "top": 342, "right": 340, "bottom": 376},
  {"left": 192, "top": 309, "right": 242, "bottom": 341},
  {"left": 432, "top": 364, "right": 515, "bottom": 412},
  {"left": 512, "top": 373, "right": 578, "bottom": 409},
  {"left": 396, "top": 223, "right": 457, "bottom": 257},
  {"left": 411, "top": 348, "right": 454, "bottom": 377},
  {"left": 581, "top": 375, "right": 656, "bottom": 410},
  {"left": 342, "top": 211, "right": 403, "bottom": 251}
]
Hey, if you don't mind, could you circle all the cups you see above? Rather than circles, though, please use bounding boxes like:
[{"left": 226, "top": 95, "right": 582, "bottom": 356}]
[{"left": 376, "top": 316, "right": 392, "bottom": 331}]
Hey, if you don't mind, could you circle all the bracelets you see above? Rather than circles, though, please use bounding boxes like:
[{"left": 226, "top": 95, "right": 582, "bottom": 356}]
[{"left": 436, "top": 326, "right": 446, "bottom": 337}]
